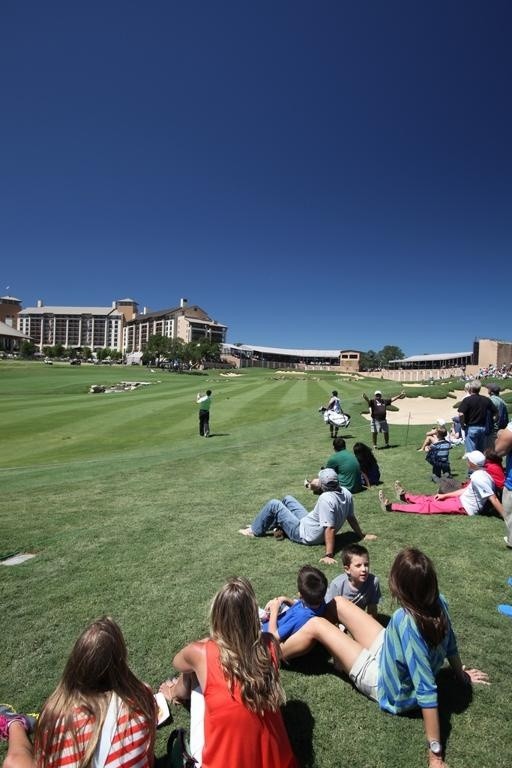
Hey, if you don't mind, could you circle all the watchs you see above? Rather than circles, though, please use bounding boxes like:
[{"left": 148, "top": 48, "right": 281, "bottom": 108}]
[
  {"left": 427, "top": 740, "right": 444, "bottom": 755},
  {"left": 325, "top": 553, "right": 333, "bottom": 558}
]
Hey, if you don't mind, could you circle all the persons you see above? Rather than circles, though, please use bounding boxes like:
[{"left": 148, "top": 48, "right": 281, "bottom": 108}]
[
  {"left": 305, "top": 438, "right": 362, "bottom": 493},
  {"left": 254, "top": 562, "right": 328, "bottom": 643},
  {"left": 362, "top": 390, "right": 405, "bottom": 449},
  {"left": 2, "top": 614, "right": 158, "bottom": 768},
  {"left": 0, "top": 705, "right": 40, "bottom": 767},
  {"left": 324, "top": 545, "right": 382, "bottom": 619},
  {"left": 326, "top": 391, "right": 343, "bottom": 437},
  {"left": 276, "top": 549, "right": 490, "bottom": 768},
  {"left": 156, "top": 577, "right": 297, "bottom": 768},
  {"left": 196, "top": 390, "right": 212, "bottom": 437},
  {"left": 238, "top": 468, "right": 378, "bottom": 565},
  {"left": 354, "top": 443, "right": 381, "bottom": 487},
  {"left": 376, "top": 364, "right": 512, "bottom": 547}
]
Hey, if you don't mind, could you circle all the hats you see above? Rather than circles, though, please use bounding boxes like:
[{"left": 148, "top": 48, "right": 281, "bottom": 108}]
[
  {"left": 318, "top": 467, "right": 339, "bottom": 492},
  {"left": 465, "top": 450, "right": 486, "bottom": 467},
  {"left": 374, "top": 391, "right": 382, "bottom": 396},
  {"left": 453, "top": 416, "right": 460, "bottom": 423},
  {"left": 438, "top": 419, "right": 446, "bottom": 427},
  {"left": 486, "top": 383, "right": 500, "bottom": 393}
]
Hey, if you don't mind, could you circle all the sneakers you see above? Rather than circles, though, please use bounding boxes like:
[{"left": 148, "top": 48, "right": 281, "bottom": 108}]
[
  {"left": 0, "top": 703, "right": 41, "bottom": 741},
  {"left": 199, "top": 431, "right": 210, "bottom": 438},
  {"left": 372, "top": 443, "right": 391, "bottom": 450},
  {"left": 274, "top": 526, "right": 285, "bottom": 541}
]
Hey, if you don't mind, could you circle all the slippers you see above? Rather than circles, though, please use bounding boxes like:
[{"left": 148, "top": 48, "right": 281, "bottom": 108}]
[
  {"left": 394, "top": 480, "right": 406, "bottom": 501},
  {"left": 378, "top": 489, "right": 392, "bottom": 512}
]
